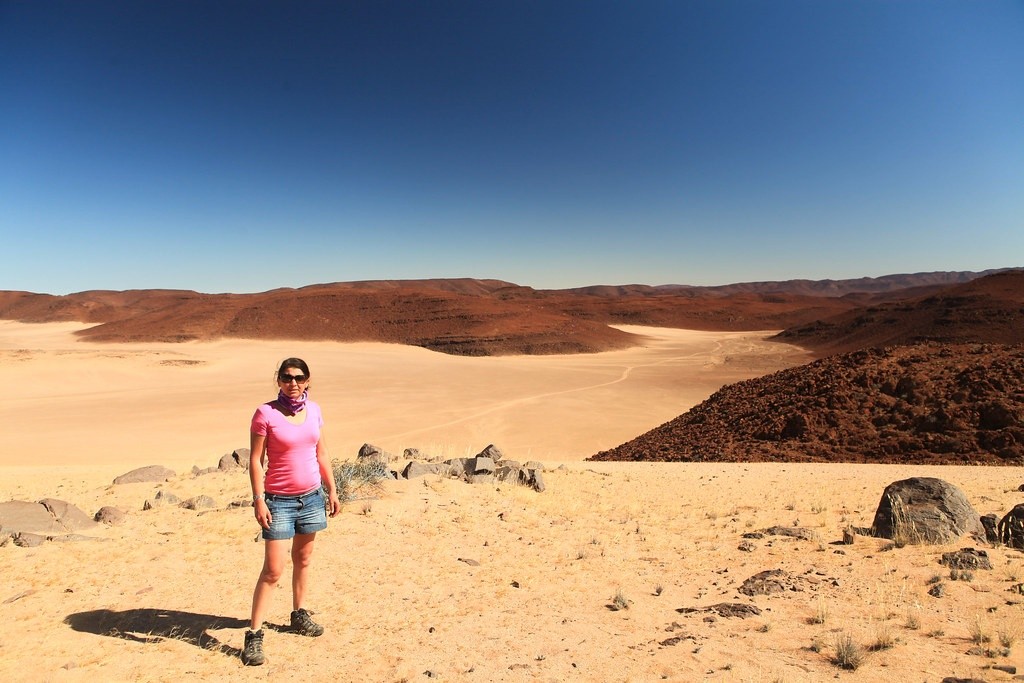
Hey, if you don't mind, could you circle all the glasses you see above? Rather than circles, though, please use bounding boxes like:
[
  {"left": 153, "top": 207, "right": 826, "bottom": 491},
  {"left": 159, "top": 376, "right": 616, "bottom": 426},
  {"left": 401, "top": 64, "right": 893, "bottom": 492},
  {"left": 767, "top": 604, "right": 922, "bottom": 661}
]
[{"left": 280, "top": 373, "right": 307, "bottom": 383}]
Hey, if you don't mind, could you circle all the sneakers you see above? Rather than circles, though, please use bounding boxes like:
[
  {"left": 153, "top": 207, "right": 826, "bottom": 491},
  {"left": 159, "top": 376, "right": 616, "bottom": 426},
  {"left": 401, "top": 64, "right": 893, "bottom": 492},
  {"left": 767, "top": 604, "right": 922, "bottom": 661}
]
[
  {"left": 242, "top": 629, "right": 264, "bottom": 666},
  {"left": 290, "top": 608, "right": 325, "bottom": 636}
]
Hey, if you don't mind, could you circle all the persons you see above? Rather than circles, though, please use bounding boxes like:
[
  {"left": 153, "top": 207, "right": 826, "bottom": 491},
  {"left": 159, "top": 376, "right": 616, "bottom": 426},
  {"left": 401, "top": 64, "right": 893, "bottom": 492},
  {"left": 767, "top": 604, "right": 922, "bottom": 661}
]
[{"left": 242, "top": 358, "right": 340, "bottom": 665}]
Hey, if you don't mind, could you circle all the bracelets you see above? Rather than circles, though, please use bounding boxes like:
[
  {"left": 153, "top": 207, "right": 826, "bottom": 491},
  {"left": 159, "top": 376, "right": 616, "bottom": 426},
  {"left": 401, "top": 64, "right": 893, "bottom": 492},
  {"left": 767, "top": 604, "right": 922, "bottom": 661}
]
[{"left": 253, "top": 495, "right": 265, "bottom": 500}]
